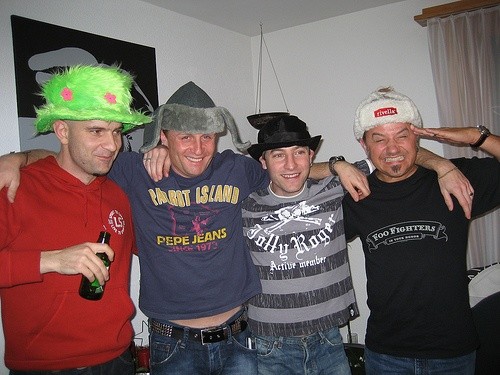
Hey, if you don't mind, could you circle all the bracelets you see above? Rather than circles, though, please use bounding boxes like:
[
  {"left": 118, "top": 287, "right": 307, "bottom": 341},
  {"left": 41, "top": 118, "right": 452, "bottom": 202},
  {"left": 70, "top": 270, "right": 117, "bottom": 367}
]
[
  {"left": 22, "top": 151, "right": 28, "bottom": 167},
  {"left": 438, "top": 167, "right": 458, "bottom": 180},
  {"left": 329, "top": 156, "right": 345, "bottom": 176}
]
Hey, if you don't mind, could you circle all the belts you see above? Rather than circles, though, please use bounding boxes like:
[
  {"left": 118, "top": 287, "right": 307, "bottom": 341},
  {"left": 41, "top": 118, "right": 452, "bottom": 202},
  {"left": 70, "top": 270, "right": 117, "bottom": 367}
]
[{"left": 148, "top": 312, "right": 249, "bottom": 343}]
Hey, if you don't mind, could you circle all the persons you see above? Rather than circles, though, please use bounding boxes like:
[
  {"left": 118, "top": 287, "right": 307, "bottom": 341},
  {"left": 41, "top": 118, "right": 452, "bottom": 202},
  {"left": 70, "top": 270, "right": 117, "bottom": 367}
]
[
  {"left": 339, "top": 85, "right": 500, "bottom": 375},
  {"left": 141, "top": 113, "right": 475, "bottom": 375},
  {"left": 1, "top": 64, "right": 155, "bottom": 375},
  {"left": 1, "top": 81, "right": 371, "bottom": 375}
]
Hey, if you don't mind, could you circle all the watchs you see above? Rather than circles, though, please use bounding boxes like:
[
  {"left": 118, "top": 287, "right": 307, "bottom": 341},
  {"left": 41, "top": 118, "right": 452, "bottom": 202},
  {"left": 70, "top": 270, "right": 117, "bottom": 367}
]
[{"left": 471, "top": 125, "right": 490, "bottom": 149}]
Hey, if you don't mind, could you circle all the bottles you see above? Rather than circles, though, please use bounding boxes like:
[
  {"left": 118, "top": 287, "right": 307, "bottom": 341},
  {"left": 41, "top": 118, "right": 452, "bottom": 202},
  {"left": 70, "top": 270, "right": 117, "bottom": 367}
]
[{"left": 79, "top": 230, "right": 111, "bottom": 301}]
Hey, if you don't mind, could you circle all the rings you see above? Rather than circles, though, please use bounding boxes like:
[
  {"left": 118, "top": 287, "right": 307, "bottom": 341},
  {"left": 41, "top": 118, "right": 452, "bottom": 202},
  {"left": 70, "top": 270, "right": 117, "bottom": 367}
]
[
  {"left": 470, "top": 193, "right": 474, "bottom": 196},
  {"left": 433, "top": 134, "right": 437, "bottom": 137},
  {"left": 142, "top": 158, "right": 151, "bottom": 161}
]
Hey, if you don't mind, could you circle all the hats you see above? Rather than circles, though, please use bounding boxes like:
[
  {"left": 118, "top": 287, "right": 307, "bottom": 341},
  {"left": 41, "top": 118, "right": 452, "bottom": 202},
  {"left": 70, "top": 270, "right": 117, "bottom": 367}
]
[
  {"left": 247, "top": 116, "right": 322, "bottom": 163},
  {"left": 138, "top": 81, "right": 252, "bottom": 153},
  {"left": 33, "top": 65, "right": 153, "bottom": 134},
  {"left": 353, "top": 91, "right": 423, "bottom": 140}
]
